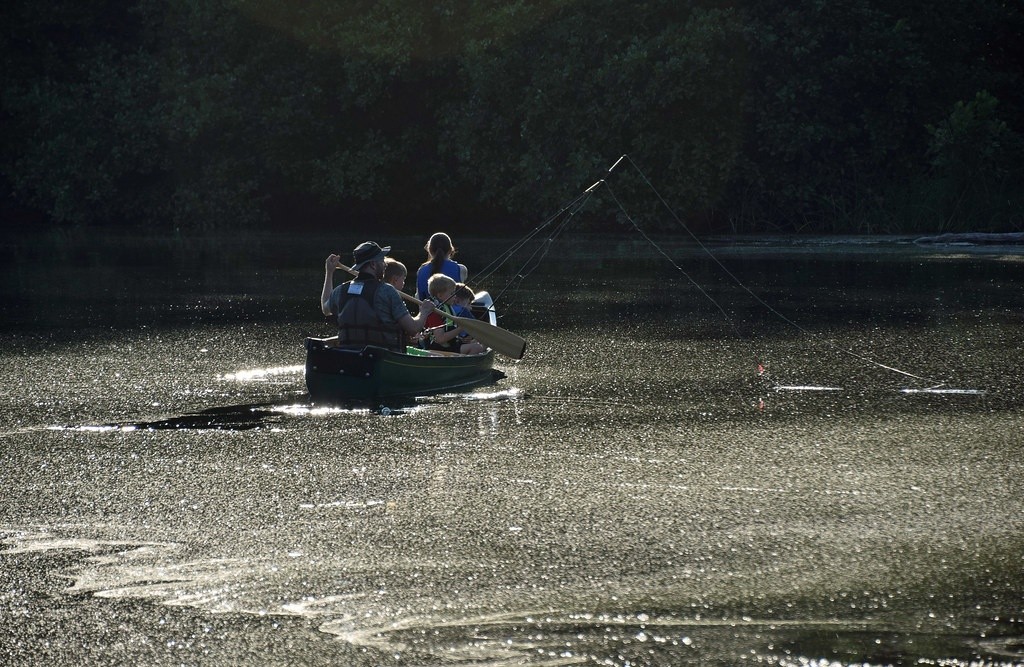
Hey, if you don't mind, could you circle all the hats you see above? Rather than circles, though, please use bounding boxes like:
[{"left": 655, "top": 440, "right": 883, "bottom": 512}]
[{"left": 348, "top": 241, "right": 391, "bottom": 271}]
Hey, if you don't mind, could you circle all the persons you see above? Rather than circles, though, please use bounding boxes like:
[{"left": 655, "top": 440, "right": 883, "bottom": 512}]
[{"left": 321, "top": 232, "right": 484, "bottom": 355}]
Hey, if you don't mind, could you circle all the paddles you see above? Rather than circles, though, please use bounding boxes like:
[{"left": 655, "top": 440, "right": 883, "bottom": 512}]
[{"left": 335, "top": 260, "right": 527, "bottom": 360}]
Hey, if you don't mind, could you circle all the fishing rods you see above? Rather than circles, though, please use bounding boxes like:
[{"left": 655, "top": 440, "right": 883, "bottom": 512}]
[{"left": 428, "top": 153, "right": 626, "bottom": 334}]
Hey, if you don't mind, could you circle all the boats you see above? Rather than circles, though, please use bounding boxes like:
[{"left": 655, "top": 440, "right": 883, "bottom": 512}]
[{"left": 302, "top": 290, "right": 498, "bottom": 403}]
